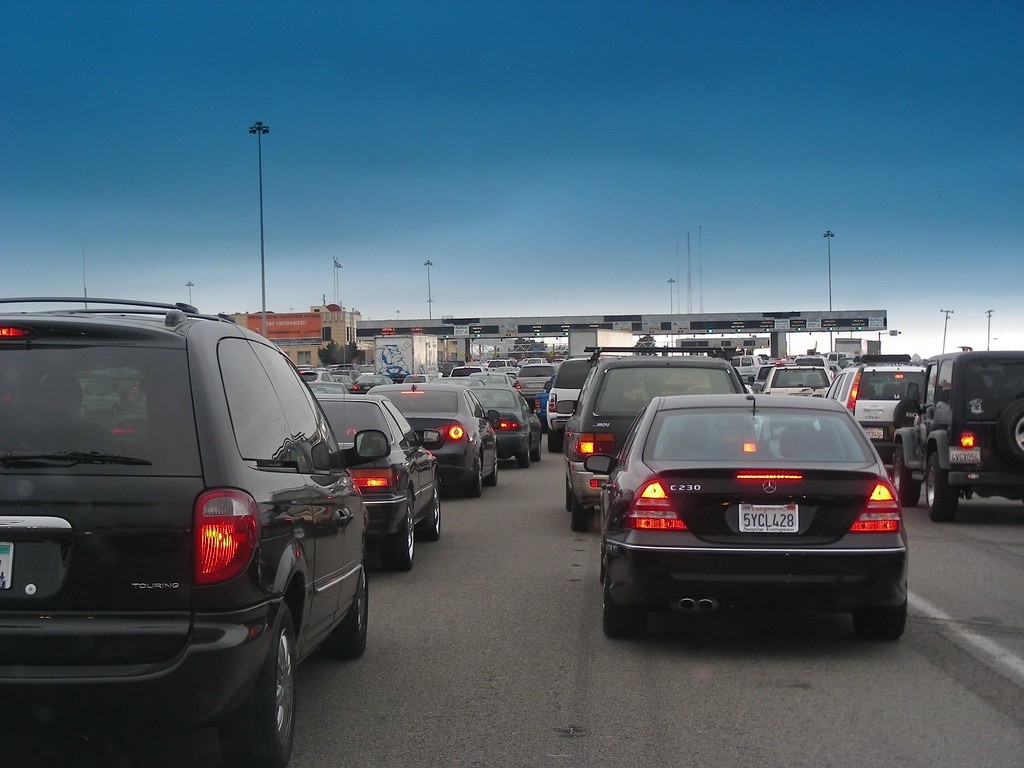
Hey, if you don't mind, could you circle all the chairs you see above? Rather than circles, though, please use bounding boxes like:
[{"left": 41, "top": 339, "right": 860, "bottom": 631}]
[
  {"left": 859, "top": 383, "right": 876, "bottom": 397},
  {"left": 778, "top": 424, "right": 836, "bottom": 461},
  {"left": 806, "top": 373, "right": 820, "bottom": 386},
  {"left": 901, "top": 380, "right": 908, "bottom": 399},
  {"left": 22, "top": 369, "right": 124, "bottom": 456}
]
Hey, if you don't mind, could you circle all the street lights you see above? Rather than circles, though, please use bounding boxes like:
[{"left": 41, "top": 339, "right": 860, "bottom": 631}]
[
  {"left": 186, "top": 282, "right": 194, "bottom": 306},
  {"left": 668, "top": 278, "right": 676, "bottom": 315},
  {"left": 940, "top": 308, "right": 953, "bottom": 354},
  {"left": 250, "top": 120, "right": 272, "bottom": 336},
  {"left": 985, "top": 308, "right": 995, "bottom": 351},
  {"left": 424, "top": 259, "right": 433, "bottom": 319},
  {"left": 823, "top": 229, "right": 834, "bottom": 313}
]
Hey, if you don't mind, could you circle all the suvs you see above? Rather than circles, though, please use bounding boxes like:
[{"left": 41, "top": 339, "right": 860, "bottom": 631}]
[
  {"left": 892, "top": 345, "right": 1024, "bottom": 523},
  {"left": 0, "top": 284, "right": 370, "bottom": 768},
  {"left": 562, "top": 344, "right": 752, "bottom": 532},
  {"left": 825, "top": 354, "right": 928, "bottom": 460}
]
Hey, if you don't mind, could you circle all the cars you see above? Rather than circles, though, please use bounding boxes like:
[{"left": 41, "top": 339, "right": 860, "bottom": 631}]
[
  {"left": 582, "top": 391, "right": 908, "bottom": 639},
  {"left": 296, "top": 356, "right": 595, "bottom": 497},
  {"left": 735, "top": 350, "right": 852, "bottom": 400},
  {"left": 314, "top": 392, "right": 441, "bottom": 572}
]
